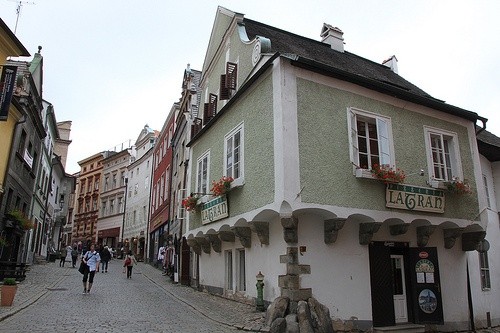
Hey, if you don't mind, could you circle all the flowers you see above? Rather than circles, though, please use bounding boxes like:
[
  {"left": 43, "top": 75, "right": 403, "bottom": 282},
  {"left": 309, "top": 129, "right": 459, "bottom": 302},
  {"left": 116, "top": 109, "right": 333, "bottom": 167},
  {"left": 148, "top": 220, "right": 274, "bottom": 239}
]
[
  {"left": 210, "top": 176, "right": 233, "bottom": 197},
  {"left": 12, "top": 212, "right": 37, "bottom": 232},
  {"left": 447, "top": 177, "right": 471, "bottom": 193},
  {"left": 372, "top": 164, "right": 406, "bottom": 183},
  {"left": 182, "top": 197, "right": 198, "bottom": 212}
]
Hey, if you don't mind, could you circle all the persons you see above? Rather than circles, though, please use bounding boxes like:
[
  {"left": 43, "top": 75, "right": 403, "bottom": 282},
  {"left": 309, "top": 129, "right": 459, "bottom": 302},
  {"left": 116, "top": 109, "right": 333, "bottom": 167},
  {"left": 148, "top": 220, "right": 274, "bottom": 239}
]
[
  {"left": 99, "top": 245, "right": 104, "bottom": 258},
  {"left": 58, "top": 246, "right": 67, "bottom": 267},
  {"left": 82, "top": 243, "right": 100, "bottom": 295},
  {"left": 101, "top": 247, "right": 111, "bottom": 273},
  {"left": 123, "top": 250, "right": 138, "bottom": 279},
  {"left": 71, "top": 246, "right": 78, "bottom": 268},
  {"left": 47, "top": 243, "right": 54, "bottom": 263}
]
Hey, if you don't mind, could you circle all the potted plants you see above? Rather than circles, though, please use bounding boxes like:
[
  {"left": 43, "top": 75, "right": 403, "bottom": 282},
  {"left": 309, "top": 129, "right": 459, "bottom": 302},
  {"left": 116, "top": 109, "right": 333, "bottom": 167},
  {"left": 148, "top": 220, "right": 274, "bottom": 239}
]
[{"left": 1, "top": 278, "right": 18, "bottom": 306}]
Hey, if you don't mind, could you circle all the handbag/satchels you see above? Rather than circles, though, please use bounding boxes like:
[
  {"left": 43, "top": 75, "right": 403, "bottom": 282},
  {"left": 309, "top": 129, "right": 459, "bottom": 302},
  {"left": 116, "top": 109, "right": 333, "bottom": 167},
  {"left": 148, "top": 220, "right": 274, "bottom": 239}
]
[
  {"left": 125, "top": 255, "right": 131, "bottom": 265},
  {"left": 79, "top": 263, "right": 90, "bottom": 274},
  {"left": 121, "top": 266, "right": 126, "bottom": 273}
]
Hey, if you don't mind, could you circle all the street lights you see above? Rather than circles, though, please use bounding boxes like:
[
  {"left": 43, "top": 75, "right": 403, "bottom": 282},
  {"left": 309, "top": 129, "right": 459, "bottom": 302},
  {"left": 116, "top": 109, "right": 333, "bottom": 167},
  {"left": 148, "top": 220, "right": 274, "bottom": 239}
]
[{"left": 121, "top": 175, "right": 128, "bottom": 248}]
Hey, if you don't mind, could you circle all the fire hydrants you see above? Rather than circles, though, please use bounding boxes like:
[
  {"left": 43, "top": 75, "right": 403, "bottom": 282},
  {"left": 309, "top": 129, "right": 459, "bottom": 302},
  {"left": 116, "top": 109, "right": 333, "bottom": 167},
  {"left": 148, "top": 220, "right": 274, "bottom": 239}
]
[{"left": 255, "top": 271, "right": 265, "bottom": 312}]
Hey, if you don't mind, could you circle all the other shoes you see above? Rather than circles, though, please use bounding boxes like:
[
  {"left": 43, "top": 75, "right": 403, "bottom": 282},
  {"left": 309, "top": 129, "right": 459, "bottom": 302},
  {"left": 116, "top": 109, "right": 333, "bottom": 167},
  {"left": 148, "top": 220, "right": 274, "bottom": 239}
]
[
  {"left": 82, "top": 288, "right": 86, "bottom": 294},
  {"left": 87, "top": 289, "right": 91, "bottom": 295}
]
